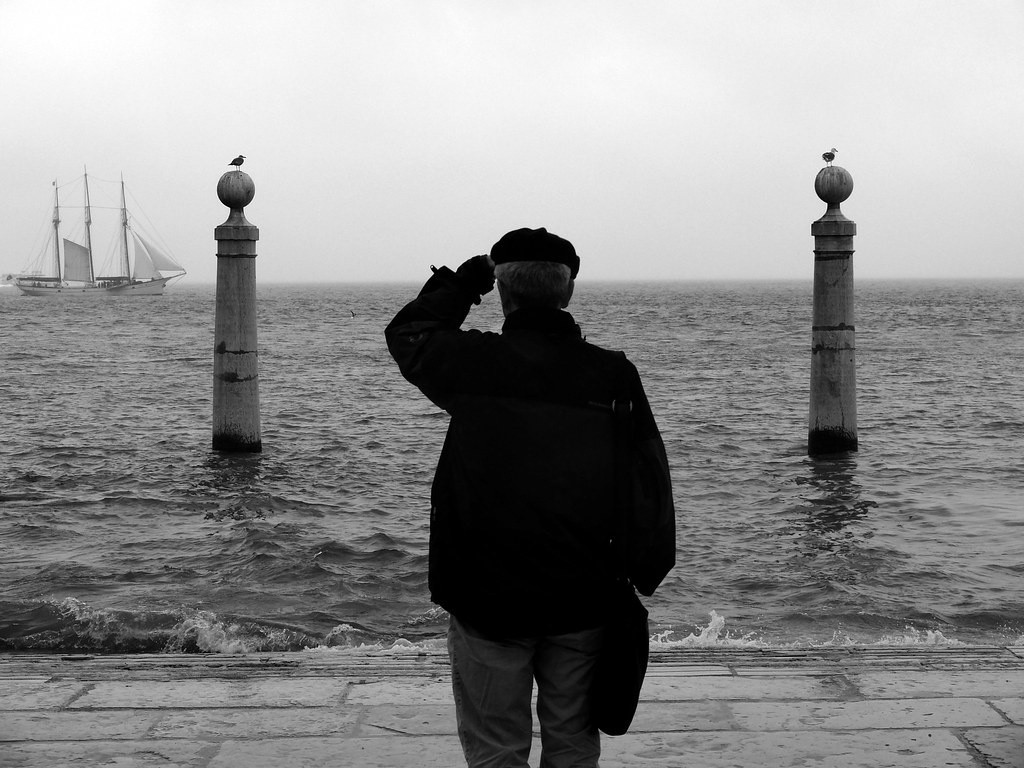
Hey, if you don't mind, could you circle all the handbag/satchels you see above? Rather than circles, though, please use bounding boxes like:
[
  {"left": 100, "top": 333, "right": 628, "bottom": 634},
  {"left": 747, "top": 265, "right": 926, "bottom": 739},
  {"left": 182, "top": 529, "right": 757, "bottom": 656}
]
[{"left": 598, "top": 572, "right": 649, "bottom": 736}]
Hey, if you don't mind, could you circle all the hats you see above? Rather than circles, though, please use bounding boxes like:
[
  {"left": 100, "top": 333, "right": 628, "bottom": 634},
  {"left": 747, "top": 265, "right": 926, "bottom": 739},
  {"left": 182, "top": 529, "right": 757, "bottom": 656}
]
[{"left": 490, "top": 227, "right": 580, "bottom": 280}]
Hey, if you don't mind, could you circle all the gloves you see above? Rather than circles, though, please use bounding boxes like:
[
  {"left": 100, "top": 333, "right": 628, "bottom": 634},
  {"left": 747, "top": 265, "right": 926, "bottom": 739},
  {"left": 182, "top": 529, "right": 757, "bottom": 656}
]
[{"left": 456, "top": 254, "right": 496, "bottom": 295}]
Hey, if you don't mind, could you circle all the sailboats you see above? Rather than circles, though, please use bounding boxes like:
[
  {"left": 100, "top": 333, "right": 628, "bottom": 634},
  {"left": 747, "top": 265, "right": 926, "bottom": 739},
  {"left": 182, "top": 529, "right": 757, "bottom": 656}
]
[{"left": 7, "top": 165, "right": 187, "bottom": 296}]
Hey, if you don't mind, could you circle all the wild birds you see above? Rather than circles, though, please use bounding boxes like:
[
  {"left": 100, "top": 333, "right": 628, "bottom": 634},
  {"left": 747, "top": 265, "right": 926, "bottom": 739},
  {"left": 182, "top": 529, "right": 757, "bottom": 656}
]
[
  {"left": 822, "top": 148, "right": 839, "bottom": 168},
  {"left": 228, "top": 155, "right": 246, "bottom": 171}
]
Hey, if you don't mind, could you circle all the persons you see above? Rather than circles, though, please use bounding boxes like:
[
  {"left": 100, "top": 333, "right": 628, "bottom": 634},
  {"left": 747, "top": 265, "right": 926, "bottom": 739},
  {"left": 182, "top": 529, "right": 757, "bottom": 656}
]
[{"left": 384, "top": 226, "right": 677, "bottom": 768}]
